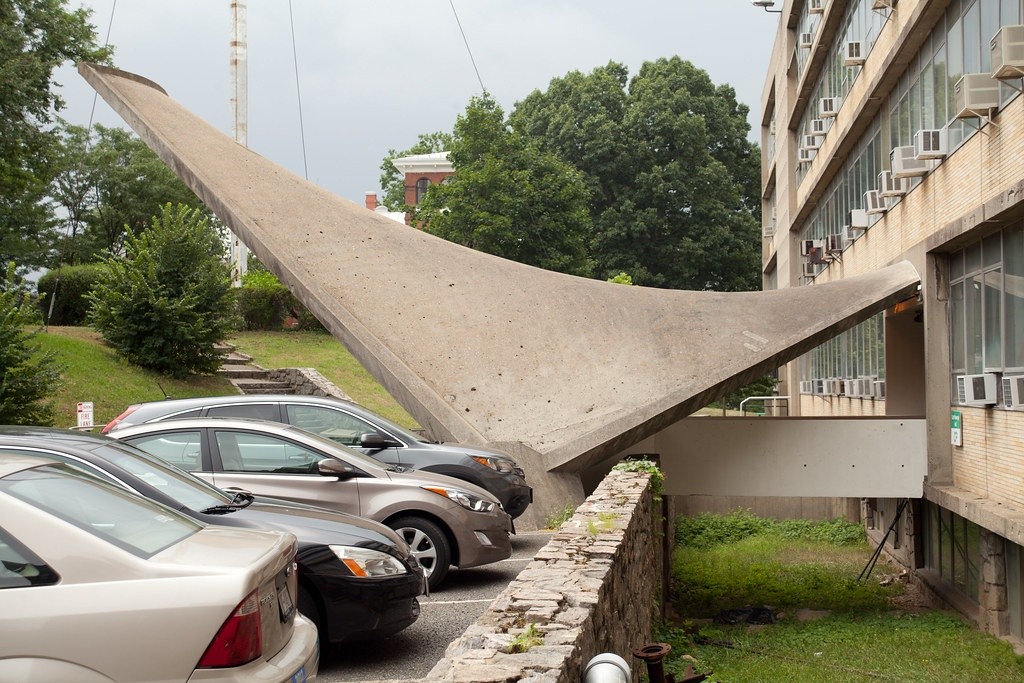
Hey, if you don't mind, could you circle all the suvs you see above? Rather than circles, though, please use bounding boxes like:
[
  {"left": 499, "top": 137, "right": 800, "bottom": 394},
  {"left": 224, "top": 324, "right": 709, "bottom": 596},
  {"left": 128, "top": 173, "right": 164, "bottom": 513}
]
[{"left": 101, "top": 382, "right": 533, "bottom": 520}]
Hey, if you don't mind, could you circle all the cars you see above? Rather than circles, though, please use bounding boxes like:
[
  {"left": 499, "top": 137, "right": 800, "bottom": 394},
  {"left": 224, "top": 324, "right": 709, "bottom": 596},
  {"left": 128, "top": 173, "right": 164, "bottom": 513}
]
[
  {"left": 100, "top": 417, "right": 516, "bottom": 593},
  {"left": 0, "top": 454, "right": 321, "bottom": 682},
  {"left": 0, "top": 424, "right": 431, "bottom": 660}
]
